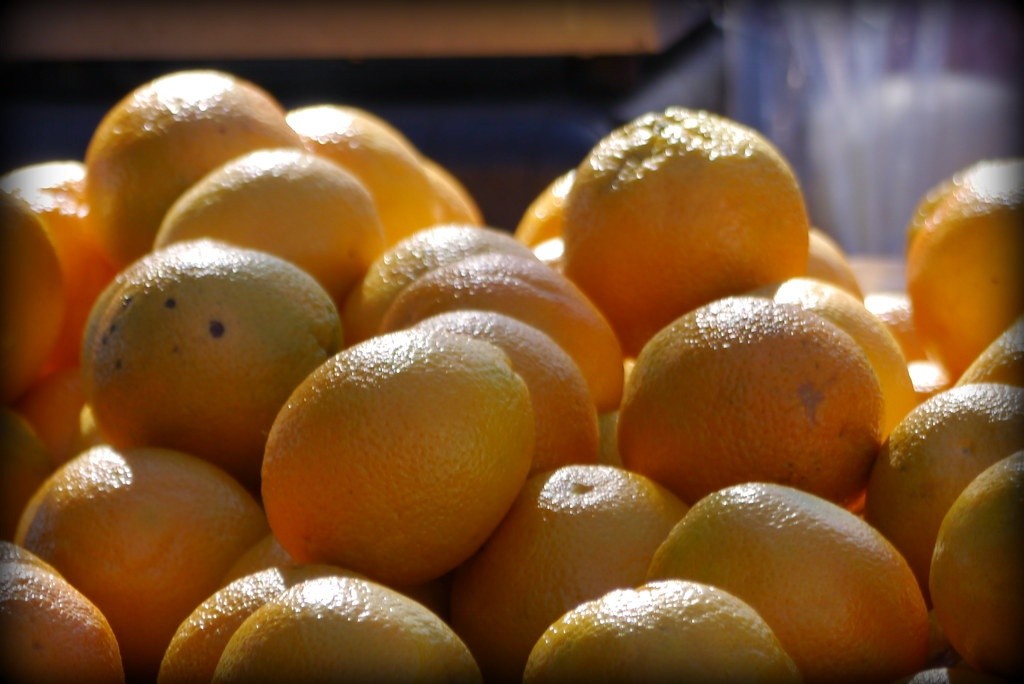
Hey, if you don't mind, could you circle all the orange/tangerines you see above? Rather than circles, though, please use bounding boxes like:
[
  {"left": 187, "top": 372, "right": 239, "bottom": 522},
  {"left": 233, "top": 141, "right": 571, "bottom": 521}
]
[{"left": 0, "top": 68, "right": 1024, "bottom": 684}]
